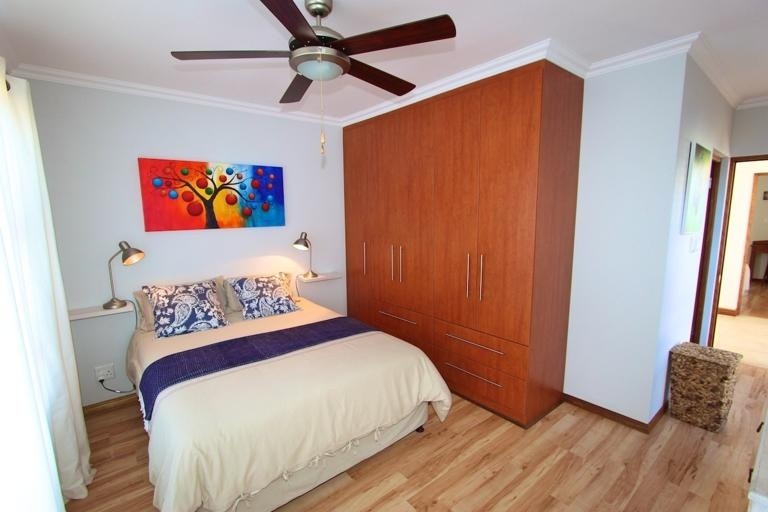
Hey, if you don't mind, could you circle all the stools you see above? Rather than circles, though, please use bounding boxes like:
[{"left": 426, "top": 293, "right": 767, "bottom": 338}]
[{"left": 668, "top": 339, "right": 745, "bottom": 434}]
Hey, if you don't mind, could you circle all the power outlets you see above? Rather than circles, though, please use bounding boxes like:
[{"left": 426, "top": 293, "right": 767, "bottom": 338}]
[{"left": 95, "top": 363, "right": 117, "bottom": 381}]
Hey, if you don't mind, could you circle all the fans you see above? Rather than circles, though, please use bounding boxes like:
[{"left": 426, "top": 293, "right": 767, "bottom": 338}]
[{"left": 170, "top": 0, "right": 459, "bottom": 105}]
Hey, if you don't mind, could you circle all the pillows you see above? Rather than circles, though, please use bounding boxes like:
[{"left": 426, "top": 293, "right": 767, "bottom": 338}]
[{"left": 132, "top": 271, "right": 298, "bottom": 338}]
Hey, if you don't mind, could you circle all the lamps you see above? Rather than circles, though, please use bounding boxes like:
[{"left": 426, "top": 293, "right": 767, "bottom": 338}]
[
  {"left": 103, "top": 240, "right": 147, "bottom": 310},
  {"left": 288, "top": 45, "right": 350, "bottom": 82},
  {"left": 293, "top": 230, "right": 321, "bottom": 280}
]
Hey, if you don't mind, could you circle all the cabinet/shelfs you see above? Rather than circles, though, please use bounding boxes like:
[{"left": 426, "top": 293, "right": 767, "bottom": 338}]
[{"left": 343, "top": 57, "right": 586, "bottom": 430}]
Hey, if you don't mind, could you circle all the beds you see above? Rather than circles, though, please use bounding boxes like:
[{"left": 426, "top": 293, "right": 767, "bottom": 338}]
[{"left": 125, "top": 296, "right": 432, "bottom": 511}]
